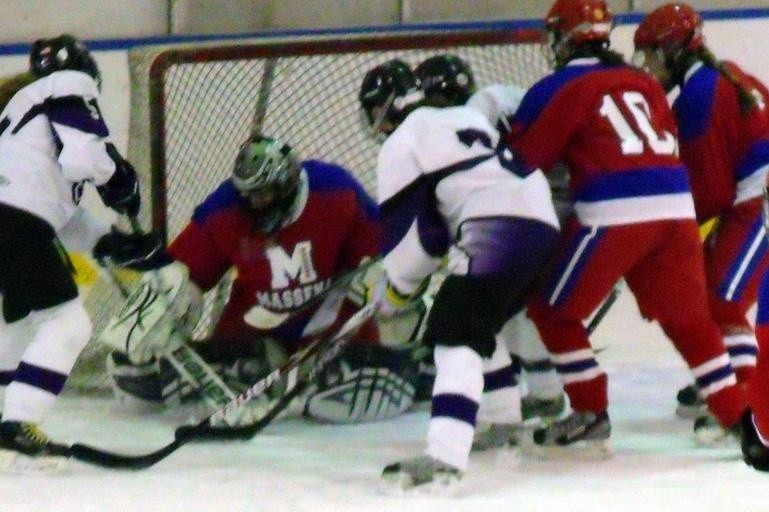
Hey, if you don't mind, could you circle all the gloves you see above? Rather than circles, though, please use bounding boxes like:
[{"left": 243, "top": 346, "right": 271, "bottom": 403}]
[
  {"left": 102, "top": 227, "right": 175, "bottom": 273},
  {"left": 97, "top": 144, "right": 140, "bottom": 220},
  {"left": 372, "top": 274, "right": 418, "bottom": 321}
]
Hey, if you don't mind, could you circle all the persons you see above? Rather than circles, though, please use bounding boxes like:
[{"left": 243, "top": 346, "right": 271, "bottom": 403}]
[
  {"left": 169, "top": 136, "right": 386, "bottom": 373},
  {"left": 0, "top": 33, "right": 168, "bottom": 467},
  {"left": 387, "top": 57, "right": 566, "bottom": 427},
  {"left": 502, "top": 0, "right": 740, "bottom": 467},
  {"left": 357, "top": 56, "right": 562, "bottom": 493},
  {"left": 742, "top": 259, "right": 769, "bottom": 471},
  {"left": 629, "top": 3, "right": 767, "bottom": 411}
]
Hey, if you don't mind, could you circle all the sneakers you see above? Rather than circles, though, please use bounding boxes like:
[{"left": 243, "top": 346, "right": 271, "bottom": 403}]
[
  {"left": 533, "top": 413, "right": 610, "bottom": 448},
  {"left": 694, "top": 413, "right": 717, "bottom": 432},
  {"left": 473, "top": 422, "right": 520, "bottom": 450},
  {"left": 677, "top": 385, "right": 703, "bottom": 407},
  {"left": 522, "top": 395, "right": 563, "bottom": 418},
  {"left": 0, "top": 422, "right": 68, "bottom": 459},
  {"left": 385, "top": 455, "right": 463, "bottom": 481}
]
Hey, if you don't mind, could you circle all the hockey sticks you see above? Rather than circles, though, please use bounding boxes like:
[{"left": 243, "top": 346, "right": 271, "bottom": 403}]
[{"left": 71, "top": 256, "right": 381, "bottom": 470}]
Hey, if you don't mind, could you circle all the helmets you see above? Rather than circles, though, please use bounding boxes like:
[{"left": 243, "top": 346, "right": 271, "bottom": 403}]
[
  {"left": 234, "top": 139, "right": 300, "bottom": 201},
  {"left": 30, "top": 34, "right": 98, "bottom": 82},
  {"left": 361, "top": 54, "right": 474, "bottom": 138},
  {"left": 634, "top": 4, "right": 701, "bottom": 77},
  {"left": 543, "top": 1, "right": 615, "bottom": 65}
]
